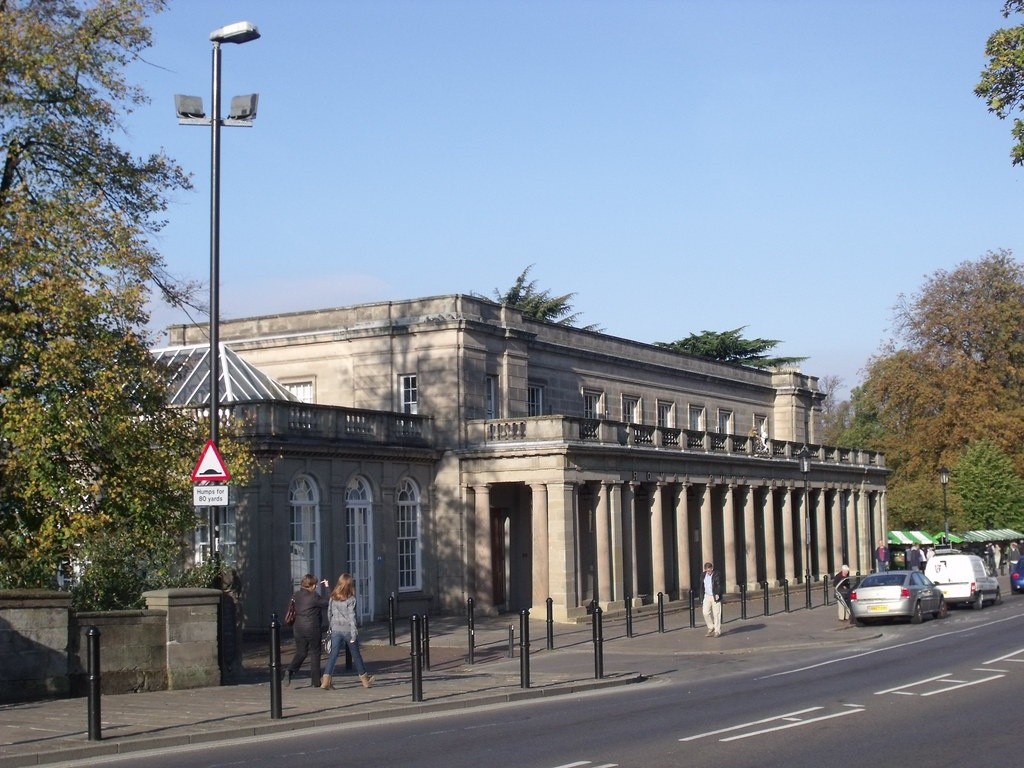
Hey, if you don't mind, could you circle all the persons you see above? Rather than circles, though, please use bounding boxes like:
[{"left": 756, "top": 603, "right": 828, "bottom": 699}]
[
  {"left": 833, "top": 564, "right": 850, "bottom": 621},
  {"left": 875, "top": 539, "right": 889, "bottom": 572},
  {"left": 987, "top": 542, "right": 1001, "bottom": 570},
  {"left": 1007, "top": 540, "right": 1024, "bottom": 574},
  {"left": 284, "top": 574, "right": 332, "bottom": 687},
  {"left": 320, "top": 573, "right": 375, "bottom": 690},
  {"left": 699, "top": 562, "right": 723, "bottom": 637},
  {"left": 905, "top": 543, "right": 934, "bottom": 571},
  {"left": 748, "top": 428, "right": 758, "bottom": 437}
]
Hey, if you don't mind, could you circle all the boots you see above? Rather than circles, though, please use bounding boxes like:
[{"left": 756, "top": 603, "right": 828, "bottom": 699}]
[
  {"left": 320, "top": 674, "right": 332, "bottom": 689},
  {"left": 359, "top": 671, "right": 376, "bottom": 688}
]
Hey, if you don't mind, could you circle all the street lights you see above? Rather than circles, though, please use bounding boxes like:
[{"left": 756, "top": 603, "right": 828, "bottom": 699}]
[
  {"left": 936, "top": 464, "right": 951, "bottom": 548},
  {"left": 171, "top": 19, "right": 264, "bottom": 587},
  {"left": 796, "top": 447, "right": 819, "bottom": 608}
]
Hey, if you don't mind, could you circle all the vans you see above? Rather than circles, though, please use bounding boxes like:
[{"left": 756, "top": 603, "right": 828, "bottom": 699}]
[{"left": 924, "top": 554, "right": 1002, "bottom": 610}]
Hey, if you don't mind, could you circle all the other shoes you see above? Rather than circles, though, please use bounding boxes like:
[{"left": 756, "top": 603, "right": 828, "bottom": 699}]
[
  {"left": 705, "top": 628, "right": 715, "bottom": 637},
  {"left": 714, "top": 632, "right": 720, "bottom": 638},
  {"left": 282, "top": 669, "right": 291, "bottom": 687},
  {"left": 311, "top": 684, "right": 319, "bottom": 688}
]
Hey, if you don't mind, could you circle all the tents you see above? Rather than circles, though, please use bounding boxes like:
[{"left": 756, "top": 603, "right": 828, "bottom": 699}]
[
  {"left": 935, "top": 529, "right": 1024, "bottom": 543},
  {"left": 887, "top": 530, "right": 938, "bottom": 545}
]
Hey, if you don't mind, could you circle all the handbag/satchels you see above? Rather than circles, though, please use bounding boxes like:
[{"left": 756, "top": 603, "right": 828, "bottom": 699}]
[
  {"left": 321, "top": 630, "right": 332, "bottom": 654},
  {"left": 285, "top": 595, "right": 295, "bottom": 625}
]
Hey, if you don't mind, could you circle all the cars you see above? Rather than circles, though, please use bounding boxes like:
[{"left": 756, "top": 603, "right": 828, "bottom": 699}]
[
  {"left": 1010, "top": 555, "right": 1024, "bottom": 596},
  {"left": 834, "top": 570, "right": 949, "bottom": 627}
]
